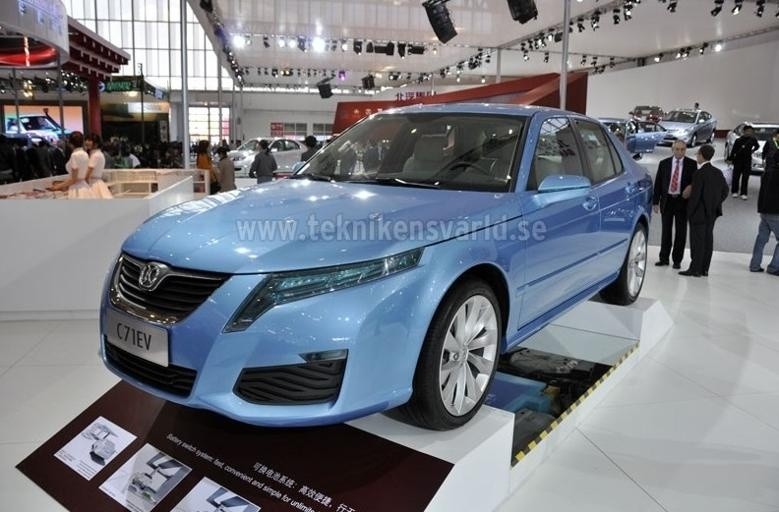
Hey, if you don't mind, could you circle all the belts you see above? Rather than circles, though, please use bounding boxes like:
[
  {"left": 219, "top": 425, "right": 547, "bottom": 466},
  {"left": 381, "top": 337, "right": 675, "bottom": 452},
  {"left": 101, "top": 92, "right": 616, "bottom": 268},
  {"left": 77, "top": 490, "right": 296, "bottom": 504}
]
[{"left": 668, "top": 194, "right": 680, "bottom": 198}]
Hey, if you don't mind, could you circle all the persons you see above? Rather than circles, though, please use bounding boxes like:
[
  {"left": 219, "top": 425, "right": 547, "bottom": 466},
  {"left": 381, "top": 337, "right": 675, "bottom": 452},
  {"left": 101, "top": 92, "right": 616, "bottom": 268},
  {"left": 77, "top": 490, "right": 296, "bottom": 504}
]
[
  {"left": 749, "top": 149, "right": 779, "bottom": 275},
  {"left": 85, "top": 134, "right": 114, "bottom": 198},
  {"left": 196, "top": 140, "right": 220, "bottom": 194},
  {"left": 0, "top": 134, "right": 182, "bottom": 185},
  {"left": 250, "top": 139, "right": 276, "bottom": 183},
  {"left": 762, "top": 128, "right": 779, "bottom": 160},
  {"left": 678, "top": 146, "right": 729, "bottom": 277},
  {"left": 300, "top": 134, "right": 379, "bottom": 175},
  {"left": 46, "top": 131, "right": 96, "bottom": 198},
  {"left": 653, "top": 138, "right": 697, "bottom": 268},
  {"left": 726, "top": 125, "right": 760, "bottom": 200},
  {"left": 190, "top": 140, "right": 241, "bottom": 152},
  {"left": 211, "top": 146, "right": 237, "bottom": 191}
]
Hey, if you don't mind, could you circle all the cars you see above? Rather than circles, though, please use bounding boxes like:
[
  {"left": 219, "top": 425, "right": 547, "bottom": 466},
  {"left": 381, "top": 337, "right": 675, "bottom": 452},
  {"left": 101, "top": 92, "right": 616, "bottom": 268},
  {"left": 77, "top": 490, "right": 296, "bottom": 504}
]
[
  {"left": 723, "top": 121, "right": 779, "bottom": 174},
  {"left": 6, "top": 114, "right": 72, "bottom": 140},
  {"left": 212, "top": 136, "right": 309, "bottom": 178},
  {"left": 578, "top": 106, "right": 717, "bottom": 161},
  {"left": 95, "top": 102, "right": 654, "bottom": 432}
]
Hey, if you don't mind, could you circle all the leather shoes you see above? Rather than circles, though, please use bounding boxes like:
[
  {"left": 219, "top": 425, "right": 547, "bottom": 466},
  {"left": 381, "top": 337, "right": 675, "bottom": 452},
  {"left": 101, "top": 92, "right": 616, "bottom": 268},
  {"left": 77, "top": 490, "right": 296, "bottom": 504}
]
[
  {"left": 655, "top": 261, "right": 669, "bottom": 266},
  {"left": 673, "top": 261, "right": 680, "bottom": 268},
  {"left": 679, "top": 269, "right": 708, "bottom": 277}
]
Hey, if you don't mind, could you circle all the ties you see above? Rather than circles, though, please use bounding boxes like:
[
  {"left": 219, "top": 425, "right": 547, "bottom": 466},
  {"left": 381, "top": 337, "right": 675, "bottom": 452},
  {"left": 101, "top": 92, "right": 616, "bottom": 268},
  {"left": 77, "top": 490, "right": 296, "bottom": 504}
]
[{"left": 671, "top": 160, "right": 679, "bottom": 192}]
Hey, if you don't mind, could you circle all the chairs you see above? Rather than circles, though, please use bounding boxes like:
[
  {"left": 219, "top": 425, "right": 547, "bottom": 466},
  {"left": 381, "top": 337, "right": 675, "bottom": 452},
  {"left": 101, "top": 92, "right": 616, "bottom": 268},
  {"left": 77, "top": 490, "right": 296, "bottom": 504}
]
[
  {"left": 402, "top": 137, "right": 462, "bottom": 175},
  {"left": 465, "top": 134, "right": 517, "bottom": 177}
]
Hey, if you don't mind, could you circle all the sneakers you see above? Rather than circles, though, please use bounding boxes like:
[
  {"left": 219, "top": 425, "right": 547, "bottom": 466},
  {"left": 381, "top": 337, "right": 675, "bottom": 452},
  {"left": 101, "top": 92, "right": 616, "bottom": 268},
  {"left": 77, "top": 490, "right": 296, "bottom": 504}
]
[
  {"left": 732, "top": 193, "right": 738, "bottom": 198},
  {"left": 740, "top": 194, "right": 748, "bottom": 200}
]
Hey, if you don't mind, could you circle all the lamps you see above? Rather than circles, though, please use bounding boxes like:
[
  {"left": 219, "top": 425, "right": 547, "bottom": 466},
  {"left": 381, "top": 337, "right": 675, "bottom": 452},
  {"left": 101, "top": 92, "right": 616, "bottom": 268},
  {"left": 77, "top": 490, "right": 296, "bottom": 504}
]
[{"left": 0, "top": 64, "right": 86, "bottom": 98}]
